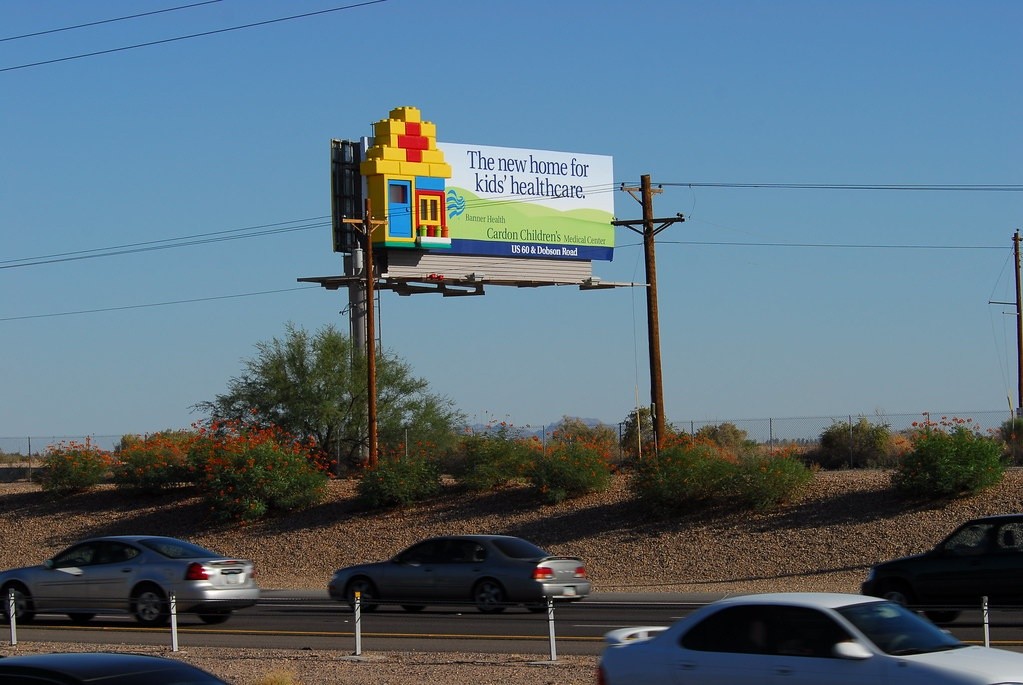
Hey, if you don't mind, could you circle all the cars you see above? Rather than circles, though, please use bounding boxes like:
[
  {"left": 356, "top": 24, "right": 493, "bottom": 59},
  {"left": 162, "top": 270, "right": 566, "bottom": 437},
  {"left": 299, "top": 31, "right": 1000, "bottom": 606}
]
[
  {"left": 861, "top": 515, "right": 1023, "bottom": 621},
  {"left": 597, "top": 591, "right": 1023, "bottom": 685},
  {"left": 1, "top": 535, "right": 261, "bottom": 626},
  {"left": 1, "top": 654, "right": 232, "bottom": 685},
  {"left": 328, "top": 535, "right": 591, "bottom": 613}
]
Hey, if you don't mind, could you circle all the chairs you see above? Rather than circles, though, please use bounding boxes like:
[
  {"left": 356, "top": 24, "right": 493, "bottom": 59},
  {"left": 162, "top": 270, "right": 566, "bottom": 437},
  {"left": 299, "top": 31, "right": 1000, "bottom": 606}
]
[{"left": 1002, "top": 530, "right": 1016, "bottom": 547}]
